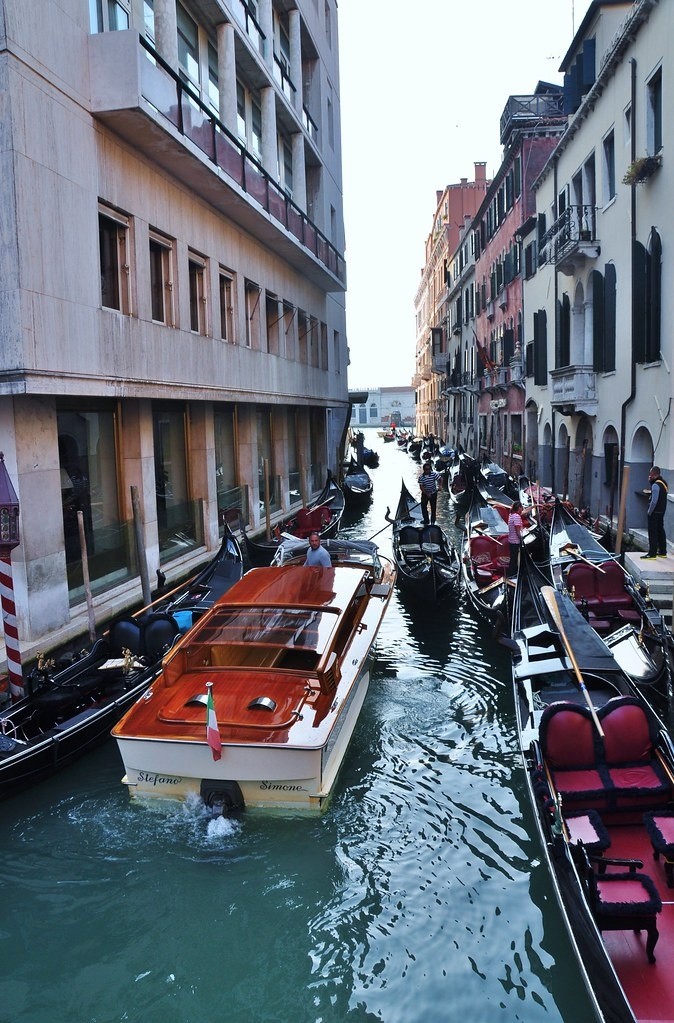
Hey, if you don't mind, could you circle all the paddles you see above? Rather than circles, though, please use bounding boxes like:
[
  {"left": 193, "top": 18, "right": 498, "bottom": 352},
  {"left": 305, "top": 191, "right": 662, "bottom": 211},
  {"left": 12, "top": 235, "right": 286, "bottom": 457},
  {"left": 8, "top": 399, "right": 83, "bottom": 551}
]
[
  {"left": 102, "top": 575, "right": 198, "bottom": 637},
  {"left": 305, "top": 496, "right": 336, "bottom": 516},
  {"left": 474, "top": 527, "right": 502, "bottom": 545},
  {"left": 535, "top": 479, "right": 547, "bottom": 556},
  {"left": 540, "top": 585, "right": 605, "bottom": 737},
  {"left": 564, "top": 548, "right": 604, "bottom": 574},
  {"left": 487, "top": 498, "right": 514, "bottom": 509},
  {"left": 368, "top": 489, "right": 440, "bottom": 542},
  {"left": 529, "top": 479, "right": 536, "bottom": 518}
]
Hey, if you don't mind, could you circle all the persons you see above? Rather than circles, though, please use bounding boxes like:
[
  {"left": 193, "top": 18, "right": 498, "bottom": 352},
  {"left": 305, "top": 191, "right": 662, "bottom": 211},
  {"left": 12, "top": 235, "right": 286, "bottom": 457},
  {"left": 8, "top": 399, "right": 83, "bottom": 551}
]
[
  {"left": 640, "top": 466, "right": 668, "bottom": 560},
  {"left": 417, "top": 463, "right": 443, "bottom": 526},
  {"left": 507, "top": 501, "right": 523, "bottom": 579},
  {"left": 302, "top": 534, "right": 332, "bottom": 568}
]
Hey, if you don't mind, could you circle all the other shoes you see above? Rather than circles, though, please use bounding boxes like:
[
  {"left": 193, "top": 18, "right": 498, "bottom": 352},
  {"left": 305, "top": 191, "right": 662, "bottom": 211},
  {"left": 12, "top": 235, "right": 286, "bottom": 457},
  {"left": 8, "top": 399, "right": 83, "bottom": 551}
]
[
  {"left": 420, "top": 520, "right": 429, "bottom": 524},
  {"left": 639, "top": 554, "right": 657, "bottom": 560},
  {"left": 656, "top": 553, "right": 668, "bottom": 558},
  {"left": 431, "top": 522, "right": 434, "bottom": 525}
]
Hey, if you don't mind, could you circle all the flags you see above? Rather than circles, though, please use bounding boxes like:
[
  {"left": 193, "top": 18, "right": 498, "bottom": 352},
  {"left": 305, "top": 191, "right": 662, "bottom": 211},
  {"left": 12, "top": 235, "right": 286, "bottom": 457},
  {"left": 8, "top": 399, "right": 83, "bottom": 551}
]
[{"left": 205, "top": 692, "right": 222, "bottom": 761}]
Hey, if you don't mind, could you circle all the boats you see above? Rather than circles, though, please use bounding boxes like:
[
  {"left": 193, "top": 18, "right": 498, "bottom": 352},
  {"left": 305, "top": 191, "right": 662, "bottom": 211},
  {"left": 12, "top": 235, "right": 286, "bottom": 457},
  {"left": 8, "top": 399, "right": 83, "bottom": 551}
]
[
  {"left": 383, "top": 431, "right": 396, "bottom": 442},
  {"left": 377, "top": 430, "right": 388, "bottom": 437},
  {"left": 547, "top": 494, "right": 674, "bottom": 759},
  {"left": 242, "top": 467, "right": 346, "bottom": 565},
  {"left": 360, "top": 446, "right": 379, "bottom": 463},
  {"left": 495, "top": 542, "right": 674, "bottom": 1023},
  {"left": 384, "top": 477, "right": 460, "bottom": 605},
  {"left": 0, "top": 518, "right": 244, "bottom": 794},
  {"left": 393, "top": 427, "right": 621, "bottom": 635},
  {"left": 109, "top": 533, "right": 397, "bottom": 816},
  {"left": 339, "top": 453, "right": 375, "bottom": 500}
]
